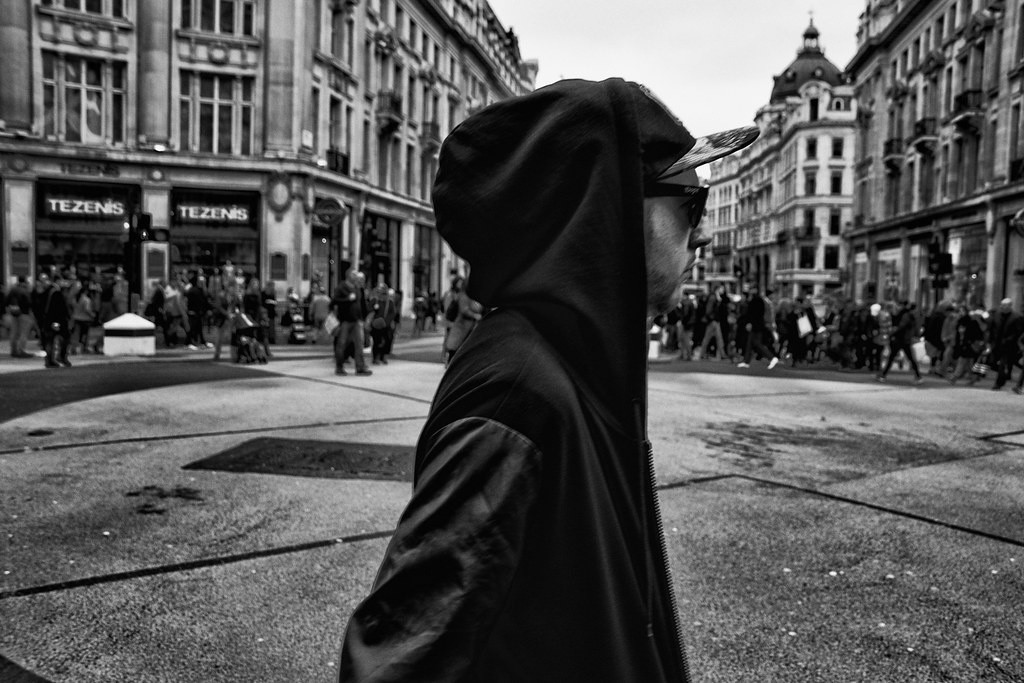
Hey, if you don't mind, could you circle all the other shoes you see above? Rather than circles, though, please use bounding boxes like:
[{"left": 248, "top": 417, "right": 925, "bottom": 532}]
[
  {"left": 737, "top": 362, "right": 749, "bottom": 368},
  {"left": 57, "top": 357, "right": 73, "bottom": 368},
  {"left": 44, "top": 360, "right": 62, "bottom": 367},
  {"left": 911, "top": 378, "right": 924, "bottom": 384},
  {"left": 10, "top": 350, "right": 35, "bottom": 358},
  {"left": 186, "top": 343, "right": 198, "bottom": 350},
  {"left": 373, "top": 358, "right": 380, "bottom": 365},
  {"left": 382, "top": 355, "right": 388, "bottom": 364},
  {"left": 355, "top": 367, "right": 373, "bottom": 375},
  {"left": 80, "top": 346, "right": 93, "bottom": 354},
  {"left": 876, "top": 375, "right": 886, "bottom": 381},
  {"left": 201, "top": 343, "right": 215, "bottom": 350},
  {"left": 334, "top": 368, "right": 346, "bottom": 375},
  {"left": 767, "top": 357, "right": 779, "bottom": 369}
]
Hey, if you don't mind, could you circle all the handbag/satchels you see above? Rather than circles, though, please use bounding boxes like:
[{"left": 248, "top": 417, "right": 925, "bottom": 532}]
[
  {"left": 372, "top": 318, "right": 386, "bottom": 331},
  {"left": 324, "top": 312, "right": 340, "bottom": 337},
  {"left": 798, "top": 315, "right": 813, "bottom": 337},
  {"left": 974, "top": 354, "right": 988, "bottom": 379},
  {"left": 445, "top": 300, "right": 459, "bottom": 323}
]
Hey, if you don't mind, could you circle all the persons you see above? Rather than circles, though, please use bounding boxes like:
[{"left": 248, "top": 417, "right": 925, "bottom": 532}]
[
  {"left": 337, "top": 76, "right": 710, "bottom": 683},
  {"left": 651, "top": 270, "right": 1024, "bottom": 395},
  {"left": 6, "top": 262, "right": 466, "bottom": 377}
]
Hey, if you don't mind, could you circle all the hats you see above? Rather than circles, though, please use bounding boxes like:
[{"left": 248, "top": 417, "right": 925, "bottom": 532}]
[
  {"left": 629, "top": 80, "right": 761, "bottom": 200},
  {"left": 870, "top": 304, "right": 882, "bottom": 316}
]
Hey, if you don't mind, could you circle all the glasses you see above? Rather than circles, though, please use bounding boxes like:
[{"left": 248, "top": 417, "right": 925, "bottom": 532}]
[{"left": 644, "top": 182, "right": 710, "bottom": 229}]
